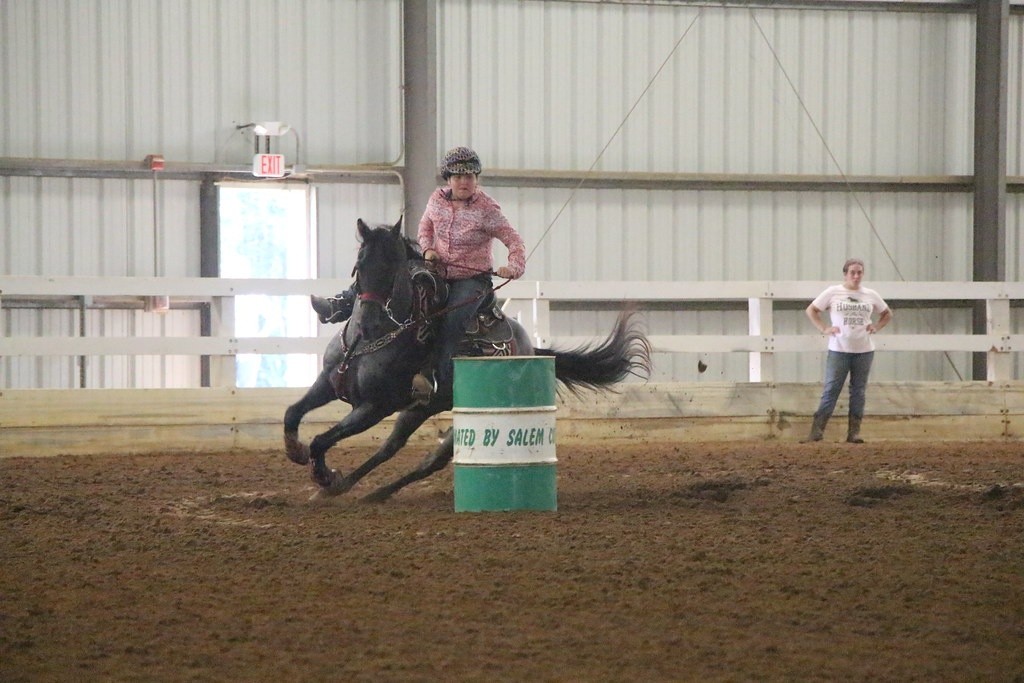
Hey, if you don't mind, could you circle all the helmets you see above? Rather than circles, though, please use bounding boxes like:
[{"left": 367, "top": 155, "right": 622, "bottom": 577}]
[{"left": 439, "top": 146, "right": 481, "bottom": 177}]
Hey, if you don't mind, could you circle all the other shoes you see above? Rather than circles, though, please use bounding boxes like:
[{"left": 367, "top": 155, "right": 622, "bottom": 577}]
[
  {"left": 413, "top": 374, "right": 432, "bottom": 406},
  {"left": 846, "top": 418, "right": 864, "bottom": 443},
  {"left": 800, "top": 416, "right": 830, "bottom": 442}
]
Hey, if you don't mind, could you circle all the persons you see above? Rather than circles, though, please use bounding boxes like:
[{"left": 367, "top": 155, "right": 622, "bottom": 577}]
[
  {"left": 416, "top": 146, "right": 527, "bottom": 411},
  {"left": 798, "top": 257, "right": 894, "bottom": 445}
]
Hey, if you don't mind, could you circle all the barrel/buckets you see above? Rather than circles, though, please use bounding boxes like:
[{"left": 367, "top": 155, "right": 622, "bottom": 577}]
[{"left": 454, "top": 354, "right": 557, "bottom": 512}]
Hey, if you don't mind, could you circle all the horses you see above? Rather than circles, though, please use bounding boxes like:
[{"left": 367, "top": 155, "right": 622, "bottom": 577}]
[{"left": 284, "top": 215, "right": 653, "bottom": 506}]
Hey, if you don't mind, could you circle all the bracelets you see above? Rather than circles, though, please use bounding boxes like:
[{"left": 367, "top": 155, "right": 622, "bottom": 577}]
[{"left": 821, "top": 324, "right": 828, "bottom": 333}]
